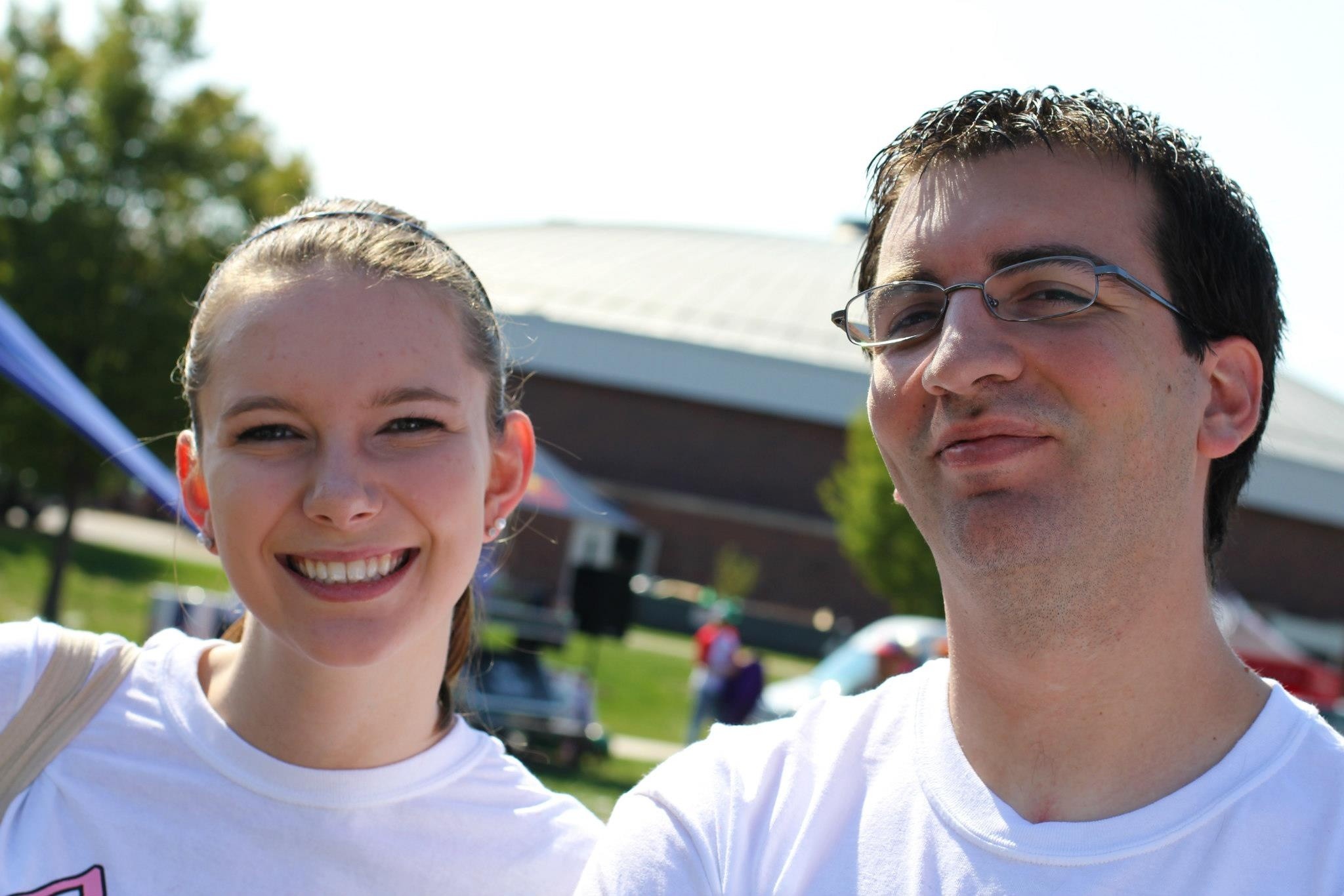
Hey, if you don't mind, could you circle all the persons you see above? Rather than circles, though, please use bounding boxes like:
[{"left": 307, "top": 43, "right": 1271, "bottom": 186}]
[
  {"left": 573, "top": 85, "right": 1344, "bottom": 896},
  {"left": 0, "top": 200, "right": 607, "bottom": 896},
  {"left": 688, "top": 610, "right": 743, "bottom": 745}
]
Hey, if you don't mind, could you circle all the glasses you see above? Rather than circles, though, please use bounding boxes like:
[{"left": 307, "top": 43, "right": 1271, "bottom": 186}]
[{"left": 832, "top": 255, "right": 1180, "bottom": 346}]
